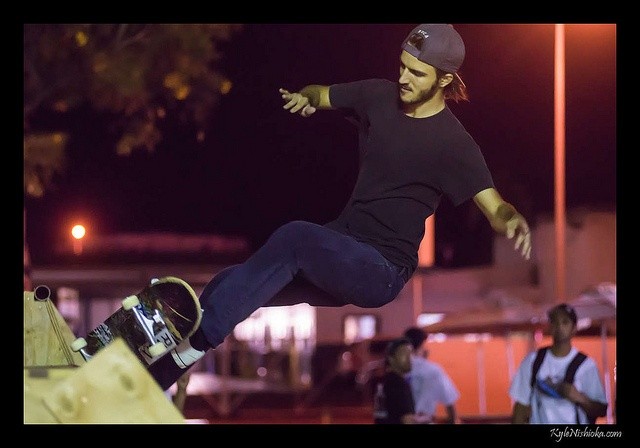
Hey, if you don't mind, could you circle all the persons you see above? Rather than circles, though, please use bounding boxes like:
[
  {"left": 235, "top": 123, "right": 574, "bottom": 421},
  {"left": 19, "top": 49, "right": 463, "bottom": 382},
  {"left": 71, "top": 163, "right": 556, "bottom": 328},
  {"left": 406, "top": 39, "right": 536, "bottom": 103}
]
[
  {"left": 149, "top": 22, "right": 532, "bottom": 390},
  {"left": 507, "top": 304, "right": 607, "bottom": 425},
  {"left": 373, "top": 337, "right": 436, "bottom": 423},
  {"left": 401, "top": 326, "right": 461, "bottom": 425}
]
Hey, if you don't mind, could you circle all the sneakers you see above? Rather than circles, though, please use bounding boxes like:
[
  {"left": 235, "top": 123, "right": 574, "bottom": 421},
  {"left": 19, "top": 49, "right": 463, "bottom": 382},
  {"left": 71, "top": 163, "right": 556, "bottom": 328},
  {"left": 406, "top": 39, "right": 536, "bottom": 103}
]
[{"left": 172, "top": 337, "right": 209, "bottom": 368}]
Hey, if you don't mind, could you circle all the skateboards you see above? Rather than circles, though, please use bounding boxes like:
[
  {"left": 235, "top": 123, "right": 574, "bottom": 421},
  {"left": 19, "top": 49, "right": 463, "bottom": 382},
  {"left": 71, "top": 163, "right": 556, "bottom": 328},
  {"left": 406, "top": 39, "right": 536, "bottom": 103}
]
[{"left": 70, "top": 275, "right": 202, "bottom": 370}]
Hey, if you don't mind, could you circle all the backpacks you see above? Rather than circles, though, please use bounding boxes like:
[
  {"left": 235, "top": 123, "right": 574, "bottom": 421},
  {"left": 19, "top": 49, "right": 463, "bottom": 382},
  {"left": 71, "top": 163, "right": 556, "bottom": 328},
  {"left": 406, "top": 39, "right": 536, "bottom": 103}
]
[{"left": 531, "top": 348, "right": 587, "bottom": 387}]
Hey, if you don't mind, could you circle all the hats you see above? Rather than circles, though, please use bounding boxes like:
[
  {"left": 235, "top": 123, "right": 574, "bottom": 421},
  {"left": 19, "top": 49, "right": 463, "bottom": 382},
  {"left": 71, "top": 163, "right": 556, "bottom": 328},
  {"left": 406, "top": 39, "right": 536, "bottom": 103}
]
[
  {"left": 385, "top": 338, "right": 412, "bottom": 363},
  {"left": 548, "top": 304, "right": 577, "bottom": 323},
  {"left": 402, "top": 24, "right": 466, "bottom": 91}
]
